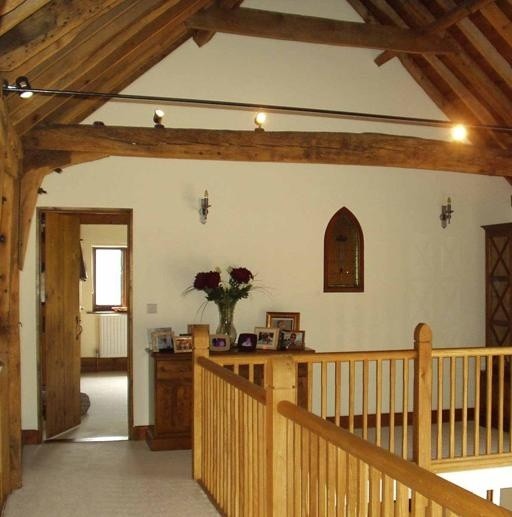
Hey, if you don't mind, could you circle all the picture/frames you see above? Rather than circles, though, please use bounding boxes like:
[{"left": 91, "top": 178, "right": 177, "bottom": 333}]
[{"left": 151, "top": 310, "right": 305, "bottom": 355}]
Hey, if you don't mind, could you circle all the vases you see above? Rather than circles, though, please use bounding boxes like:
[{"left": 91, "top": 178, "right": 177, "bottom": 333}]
[{"left": 216, "top": 301, "right": 237, "bottom": 346}]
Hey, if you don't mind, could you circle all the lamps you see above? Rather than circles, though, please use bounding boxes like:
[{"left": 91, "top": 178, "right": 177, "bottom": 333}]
[
  {"left": 153, "top": 109, "right": 165, "bottom": 127},
  {"left": 3, "top": 76, "right": 34, "bottom": 100}
]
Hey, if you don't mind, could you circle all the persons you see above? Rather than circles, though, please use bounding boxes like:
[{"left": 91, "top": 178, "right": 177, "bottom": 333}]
[{"left": 290, "top": 334, "right": 297, "bottom": 345}]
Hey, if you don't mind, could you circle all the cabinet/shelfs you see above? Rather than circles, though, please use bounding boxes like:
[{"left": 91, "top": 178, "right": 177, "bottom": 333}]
[{"left": 145, "top": 348, "right": 315, "bottom": 452}]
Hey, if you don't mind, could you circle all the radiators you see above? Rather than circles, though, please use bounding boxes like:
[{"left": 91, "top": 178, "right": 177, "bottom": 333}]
[{"left": 97, "top": 313, "right": 128, "bottom": 357}]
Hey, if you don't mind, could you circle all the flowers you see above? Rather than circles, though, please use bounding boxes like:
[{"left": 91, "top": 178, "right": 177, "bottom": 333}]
[{"left": 179, "top": 265, "right": 275, "bottom": 335}]
